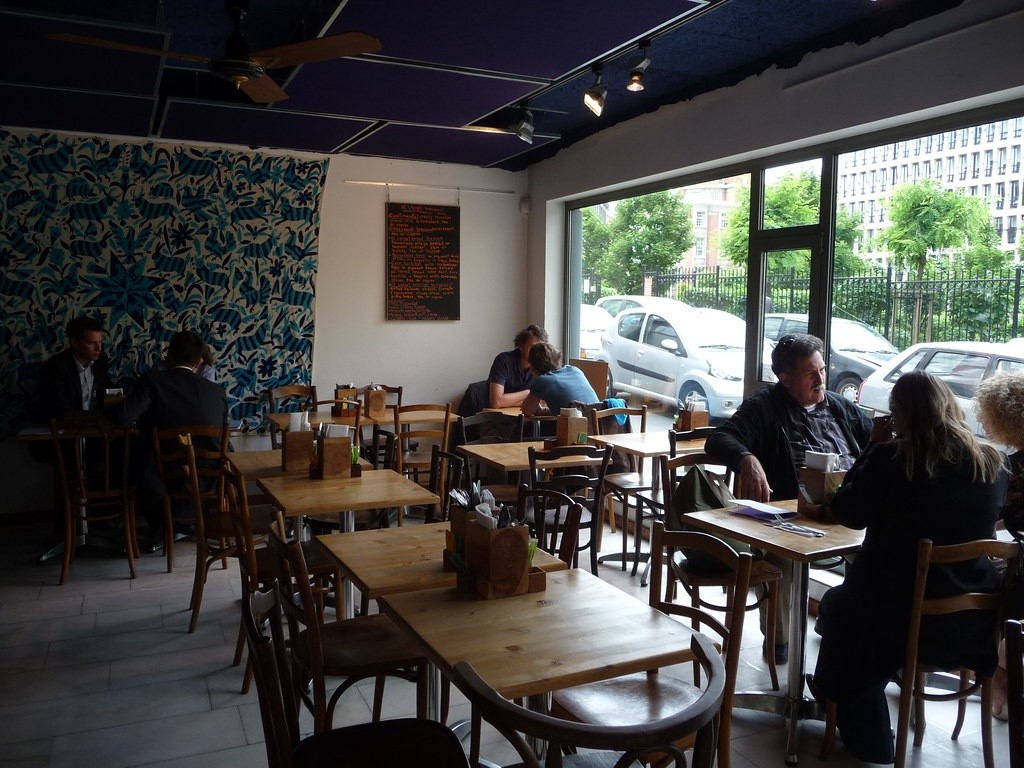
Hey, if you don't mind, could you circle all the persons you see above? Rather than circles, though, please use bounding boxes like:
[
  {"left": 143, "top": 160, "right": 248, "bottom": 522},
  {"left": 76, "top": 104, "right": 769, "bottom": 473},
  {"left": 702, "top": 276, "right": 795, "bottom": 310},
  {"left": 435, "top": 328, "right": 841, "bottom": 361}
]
[
  {"left": 481, "top": 324, "right": 545, "bottom": 409},
  {"left": 41, "top": 317, "right": 127, "bottom": 528},
  {"left": 705, "top": 334, "right": 873, "bottom": 666},
  {"left": 521, "top": 343, "right": 600, "bottom": 417},
  {"left": 138, "top": 332, "right": 229, "bottom": 542},
  {"left": 807, "top": 373, "right": 1024, "bottom": 763}
]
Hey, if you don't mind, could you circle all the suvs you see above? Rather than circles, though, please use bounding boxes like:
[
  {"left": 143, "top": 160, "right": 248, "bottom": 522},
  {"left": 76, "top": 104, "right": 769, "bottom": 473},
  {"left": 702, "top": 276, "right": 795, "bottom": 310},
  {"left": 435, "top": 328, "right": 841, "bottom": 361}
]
[{"left": 856, "top": 340, "right": 1024, "bottom": 457}]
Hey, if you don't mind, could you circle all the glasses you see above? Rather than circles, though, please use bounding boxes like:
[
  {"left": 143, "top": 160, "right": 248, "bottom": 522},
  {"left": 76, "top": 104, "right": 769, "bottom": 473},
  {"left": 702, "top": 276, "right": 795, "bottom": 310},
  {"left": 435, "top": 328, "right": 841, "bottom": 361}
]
[{"left": 889, "top": 402, "right": 899, "bottom": 411}]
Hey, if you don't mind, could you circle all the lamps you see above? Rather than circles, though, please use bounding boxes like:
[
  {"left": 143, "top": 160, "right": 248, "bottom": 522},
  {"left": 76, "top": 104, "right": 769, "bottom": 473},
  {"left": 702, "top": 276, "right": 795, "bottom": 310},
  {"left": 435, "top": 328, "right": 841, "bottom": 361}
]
[
  {"left": 625, "top": 39, "right": 652, "bottom": 92},
  {"left": 582, "top": 66, "right": 609, "bottom": 117},
  {"left": 509, "top": 101, "right": 534, "bottom": 145}
]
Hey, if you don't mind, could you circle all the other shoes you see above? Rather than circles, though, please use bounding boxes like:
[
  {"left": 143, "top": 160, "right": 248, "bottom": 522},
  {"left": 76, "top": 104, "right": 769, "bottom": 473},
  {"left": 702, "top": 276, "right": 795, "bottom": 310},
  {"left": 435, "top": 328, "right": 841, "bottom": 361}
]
[
  {"left": 763, "top": 636, "right": 788, "bottom": 664},
  {"left": 991, "top": 691, "right": 1009, "bottom": 721},
  {"left": 806, "top": 674, "right": 827, "bottom": 708},
  {"left": 174, "top": 520, "right": 194, "bottom": 535},
  {"left": 143, "top": 526, "right": 163, "bottom": 538}
]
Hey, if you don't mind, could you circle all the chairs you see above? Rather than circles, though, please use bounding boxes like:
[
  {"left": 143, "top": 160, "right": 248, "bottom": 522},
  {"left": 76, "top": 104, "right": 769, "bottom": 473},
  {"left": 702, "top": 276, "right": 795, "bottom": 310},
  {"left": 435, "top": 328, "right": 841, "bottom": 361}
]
[{"left": 50, "top": 384, "right": 1024, "bottom": 768}]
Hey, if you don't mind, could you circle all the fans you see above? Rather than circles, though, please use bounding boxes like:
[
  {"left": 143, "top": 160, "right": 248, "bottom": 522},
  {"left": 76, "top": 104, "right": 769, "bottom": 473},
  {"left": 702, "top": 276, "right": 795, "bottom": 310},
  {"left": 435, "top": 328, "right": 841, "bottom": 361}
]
[{"left": 42, "top": 0, "right": 382, "bottom": 103}]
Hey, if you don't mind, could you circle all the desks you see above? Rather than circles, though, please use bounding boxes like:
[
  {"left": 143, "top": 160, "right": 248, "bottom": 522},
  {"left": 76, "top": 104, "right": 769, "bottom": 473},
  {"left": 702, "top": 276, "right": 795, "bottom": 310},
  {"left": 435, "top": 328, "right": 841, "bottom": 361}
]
[
  {"left": 224, "top": 449, "right": 373, "bottom": 631},
  {"left": 360, "top": 409, "right": 464, "bottom": 524},
  {"left": 681, "top": 497, "right": 868, "bottom": 768},
  {"left": 265, "top": 412, "right": 375, "bottom": 440},
  {"left": 375, "top": 568, "right": 722, "bottom": 768},
  {"left": 17, "top": 415, "right": 245, "bottom": 562},
  {"left": 482, "top": 406, "right": 558, "bottom": 508},
  {"left": 455, "top": 441, "right": 612, "bottom": 509},
  {"left": 586, "top": 430, "right": 707, "bottom": 588},
  {"left": 313, "top": 521, "right": 567, "bottom": 768},
  {"left": 256, "top": 468, "right": 441, "bottom": 622}
]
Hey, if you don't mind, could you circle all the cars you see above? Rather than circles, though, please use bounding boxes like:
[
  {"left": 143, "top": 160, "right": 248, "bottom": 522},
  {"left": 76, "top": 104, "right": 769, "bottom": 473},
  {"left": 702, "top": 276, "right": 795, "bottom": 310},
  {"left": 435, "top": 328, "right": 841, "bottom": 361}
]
[
  {"left": 757, "top": 314, "right": 903, "bottom": 406},
  {"left": 595, "top": 295, "right": 689, "bottom": 319},
  {"left": 595, "top": 306, "right": 779, "bottom": 426},
  {"left": 580, "top": 304, "right": 615, "bottom": 359}
]
